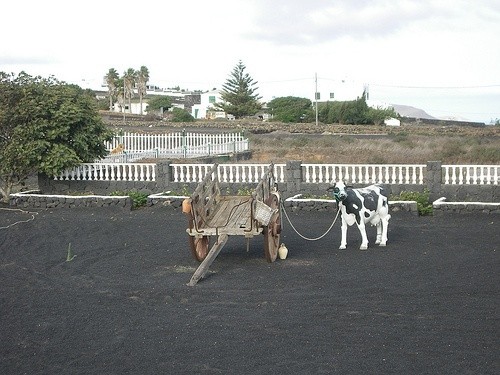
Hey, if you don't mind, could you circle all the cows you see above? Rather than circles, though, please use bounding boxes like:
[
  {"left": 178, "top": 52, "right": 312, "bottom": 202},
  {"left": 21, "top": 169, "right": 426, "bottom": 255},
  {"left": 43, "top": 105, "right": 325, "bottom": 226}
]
[{"left": 327, "top": 180, "right": 392, "bottom": 251}]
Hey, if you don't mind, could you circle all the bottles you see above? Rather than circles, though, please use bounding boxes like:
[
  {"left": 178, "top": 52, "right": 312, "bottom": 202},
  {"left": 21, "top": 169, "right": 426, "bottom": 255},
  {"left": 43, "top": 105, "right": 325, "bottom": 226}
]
[{"left": 279, "top": 243, "right": 288, "bottom": 259}]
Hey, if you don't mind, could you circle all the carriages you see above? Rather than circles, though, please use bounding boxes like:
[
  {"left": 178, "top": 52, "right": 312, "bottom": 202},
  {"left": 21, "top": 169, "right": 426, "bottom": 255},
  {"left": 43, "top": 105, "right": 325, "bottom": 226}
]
[{"left": 176, "top": 163, "right": 285, "bottom": 288}]
